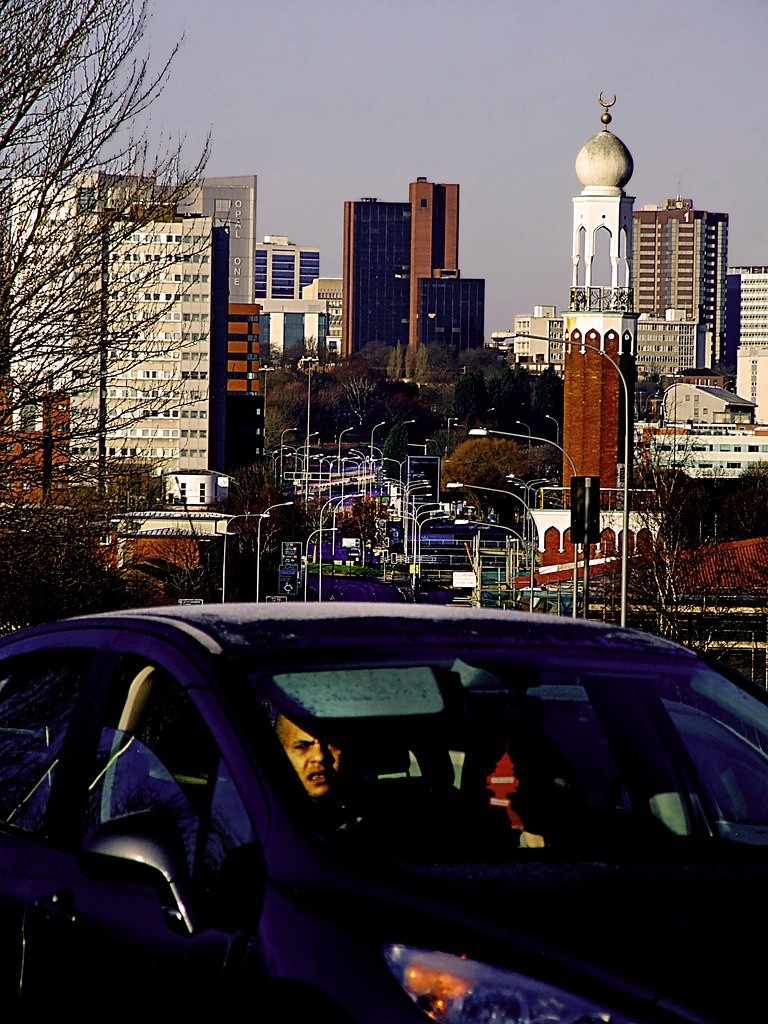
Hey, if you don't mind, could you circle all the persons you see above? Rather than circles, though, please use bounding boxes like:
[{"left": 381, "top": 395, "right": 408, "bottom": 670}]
[{"left": 196, "top": 707, "right": 344, "bottom": 920}]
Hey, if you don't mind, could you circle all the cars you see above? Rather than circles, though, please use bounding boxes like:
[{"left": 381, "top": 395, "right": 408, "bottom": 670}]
[{"left": 0, "top": 599, "right": 767, "bottom": 1024}]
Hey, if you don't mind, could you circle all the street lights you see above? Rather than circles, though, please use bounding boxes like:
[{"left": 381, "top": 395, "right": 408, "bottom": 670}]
[
  {"left": 221, "top": 512, "right": 271, "bottom": 603},
  {"left": 304, "top": 528, "right": 339, "bottom": 603},
  {"left": 489, "top": 329, "right": 631, "bottom": 628},
  {"left": 301, "top": 356, "right": 319, "bottom": 471},
  {"left": 424, "top": 438, "right": 438, "bottom": 455},
  {"left": 447, "top": 417, "right": 459, "bottom": 449},
  {"left": 545, "top": 414, "right": 561, "bottom": 445},
  {"left": 516, "top": 420, "right": 532, "bottom": 465},
  {"left": 454, "top": 518, "right": 529, "bottom": 573},
  {"left": 665, "top": 372, "right": 685, "bottom": 470},
  {"left": 445, "top": 481, "right": 536, "bottom": 613},
  {"left": 258, "top": 364, "right": 275, "bottom": 416},
  {"left": 255, "top": 501, "right": 295, "bottom": 603},
  {"left": 467, "top": 428, "right": 580, "bottom": 619},
  {"left": 262, "top": 418, "right": 558, "bottom": 599},
  {"left": 318, "top": 494, "right": 363, "bottom": 603}
]
